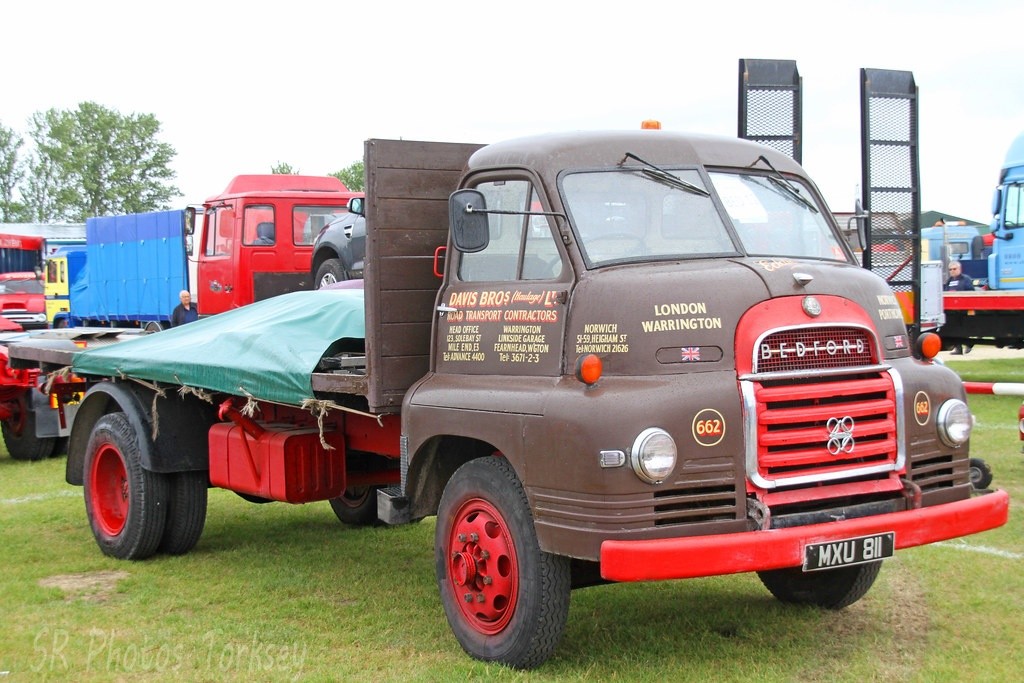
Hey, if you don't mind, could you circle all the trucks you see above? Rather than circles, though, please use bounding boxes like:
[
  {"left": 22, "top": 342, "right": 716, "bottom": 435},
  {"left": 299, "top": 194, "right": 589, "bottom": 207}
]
[{"left": 0, "top": 57, "right": 1024, "bottom": 673}]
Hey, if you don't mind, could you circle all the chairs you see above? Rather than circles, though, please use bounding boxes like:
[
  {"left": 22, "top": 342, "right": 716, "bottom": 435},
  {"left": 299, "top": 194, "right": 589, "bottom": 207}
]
[{"left": 254, "top": 222, "right": 275, "bottom": 245}]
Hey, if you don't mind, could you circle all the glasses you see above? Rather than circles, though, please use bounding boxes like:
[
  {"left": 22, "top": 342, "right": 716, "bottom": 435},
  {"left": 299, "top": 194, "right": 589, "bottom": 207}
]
[{"left": 948, "top": 267, "right": 960, "bottom": 271}]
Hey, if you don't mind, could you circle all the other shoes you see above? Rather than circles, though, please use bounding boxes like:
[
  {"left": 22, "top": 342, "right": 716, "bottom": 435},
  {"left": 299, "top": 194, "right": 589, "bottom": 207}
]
[
  {"left": 963, "top": 345, "right": 971, "bottom": 354},
  {"left": 949, "top": 347, "right": 963, "bottom": 355}
]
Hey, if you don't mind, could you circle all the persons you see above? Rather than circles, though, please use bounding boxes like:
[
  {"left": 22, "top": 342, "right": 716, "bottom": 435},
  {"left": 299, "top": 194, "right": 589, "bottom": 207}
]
[
  {"left": 171, "top": 290, "right": 199, "bottom": 327},
  {"left": 944, "top": 260, "right": 975, "bottom": 355}
]
